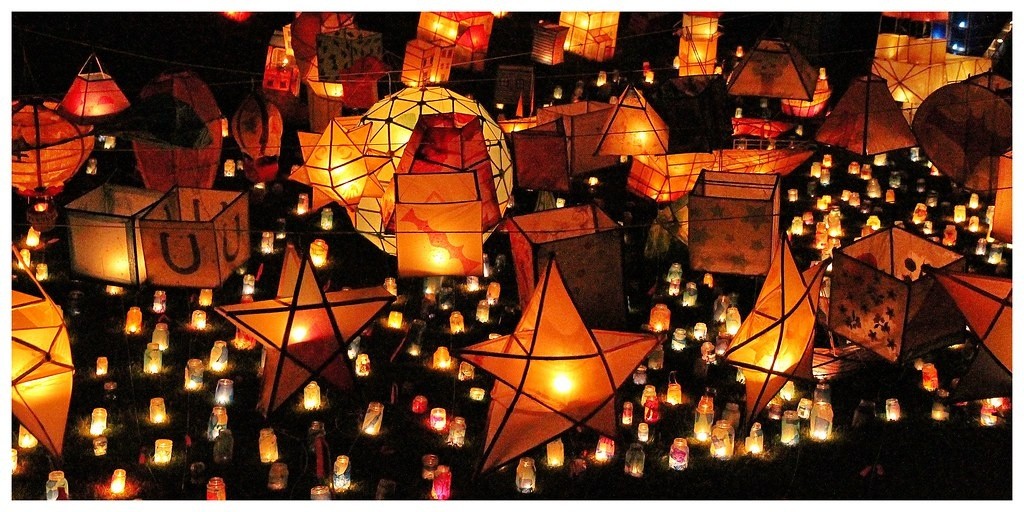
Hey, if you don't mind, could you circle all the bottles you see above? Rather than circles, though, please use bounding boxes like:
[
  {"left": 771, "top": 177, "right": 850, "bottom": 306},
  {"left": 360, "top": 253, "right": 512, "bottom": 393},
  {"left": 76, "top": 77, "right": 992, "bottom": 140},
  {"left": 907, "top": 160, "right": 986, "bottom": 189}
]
[{"left": 12, "top": 44, "right": 1012, "bottom": 500}]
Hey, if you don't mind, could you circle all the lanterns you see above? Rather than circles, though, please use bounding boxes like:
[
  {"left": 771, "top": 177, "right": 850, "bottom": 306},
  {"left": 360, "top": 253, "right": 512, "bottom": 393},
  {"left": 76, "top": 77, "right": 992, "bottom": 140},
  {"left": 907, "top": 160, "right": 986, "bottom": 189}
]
[
  {"left": 449, "top": 248, "right": 668, "bottom": 480},
  {"left": 12, "top": 12, "right": 1012, "bottom": 500}
]
